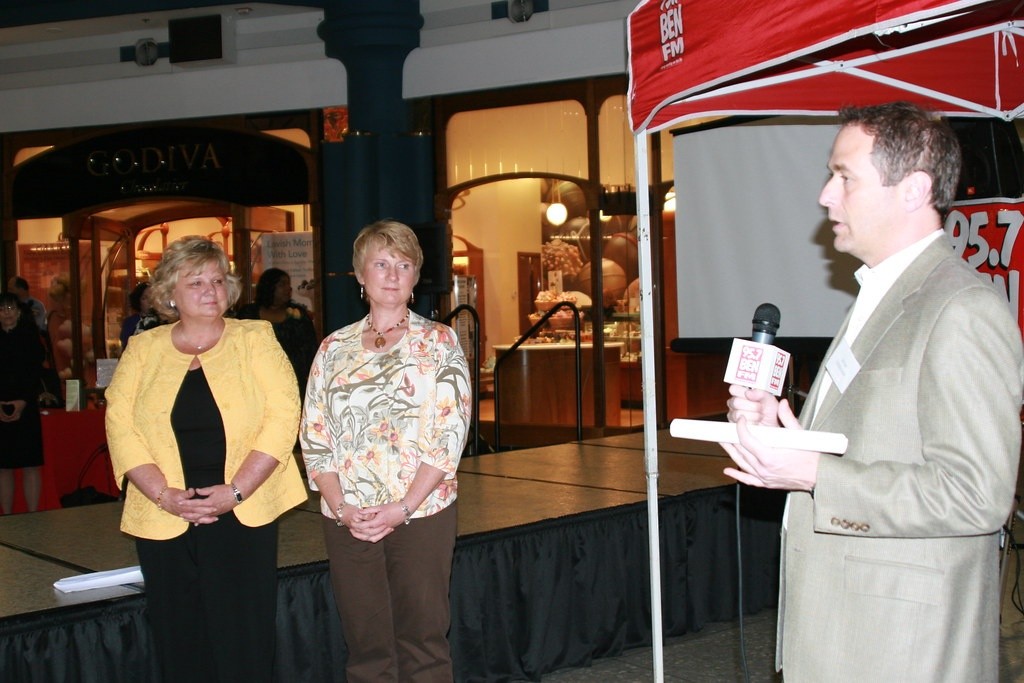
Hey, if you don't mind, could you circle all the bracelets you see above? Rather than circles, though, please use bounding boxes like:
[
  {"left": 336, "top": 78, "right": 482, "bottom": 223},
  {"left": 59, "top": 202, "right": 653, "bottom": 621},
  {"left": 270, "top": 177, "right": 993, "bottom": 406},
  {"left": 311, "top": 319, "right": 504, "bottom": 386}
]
[
  {"left": 336, "top": 502, "right": 346, "bottom": 527},
  {"left": 400, "top": 498, "right": 411, "bottom": 524},
  {"left": 157, "top": 486, "right": 167, "bottom": 511}
]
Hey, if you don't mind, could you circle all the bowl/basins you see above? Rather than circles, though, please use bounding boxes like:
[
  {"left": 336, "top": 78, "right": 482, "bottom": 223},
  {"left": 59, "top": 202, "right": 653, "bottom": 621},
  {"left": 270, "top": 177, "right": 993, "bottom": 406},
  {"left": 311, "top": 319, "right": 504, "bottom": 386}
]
[
  {"left": 527, "top": 317, "right": 549, "bottom": 327},
  {"left": 550, "top": 317, "right": 582, "bottom": 330},
  {"left": 535, "top": 300, "right": 576, "bottom": 312}
]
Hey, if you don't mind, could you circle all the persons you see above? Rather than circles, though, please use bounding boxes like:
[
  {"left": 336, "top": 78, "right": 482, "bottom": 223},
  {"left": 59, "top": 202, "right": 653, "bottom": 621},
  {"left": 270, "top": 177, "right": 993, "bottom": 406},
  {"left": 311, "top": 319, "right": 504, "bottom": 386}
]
[
  {"left": 298, "top": 221, "right": 472, "bottom": 683},
  {"left": 0, "top": 277, "right": 62, "bottom": 514},
  {"left": 718, "top": 98, "right": 1024, "bottom": 683},
  {"left": 120, "top": 281, "right": 170, "bottom": 351},
  {"left": 104, "top": 234, "right": 308, "bottom": 683},
  {"left": 582, "top": 290, "right": 622, "bottom": 321},
  {"left": 237, "top": 267, "right": 319, "bottom": 404}
]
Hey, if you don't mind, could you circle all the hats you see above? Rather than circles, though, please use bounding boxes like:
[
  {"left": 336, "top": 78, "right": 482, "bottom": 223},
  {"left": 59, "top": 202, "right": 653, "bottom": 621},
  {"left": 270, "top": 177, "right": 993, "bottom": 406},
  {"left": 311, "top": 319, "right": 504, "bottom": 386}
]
[{"left": 129, "top": 281, "right": 154, "bottom": 312}]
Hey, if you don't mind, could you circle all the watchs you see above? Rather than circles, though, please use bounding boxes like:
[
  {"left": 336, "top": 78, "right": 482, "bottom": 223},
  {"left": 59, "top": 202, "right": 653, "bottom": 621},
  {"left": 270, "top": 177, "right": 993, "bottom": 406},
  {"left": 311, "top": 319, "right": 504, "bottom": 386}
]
[{"left": 232, "top": 483, "right": 242, "bottom": 504}]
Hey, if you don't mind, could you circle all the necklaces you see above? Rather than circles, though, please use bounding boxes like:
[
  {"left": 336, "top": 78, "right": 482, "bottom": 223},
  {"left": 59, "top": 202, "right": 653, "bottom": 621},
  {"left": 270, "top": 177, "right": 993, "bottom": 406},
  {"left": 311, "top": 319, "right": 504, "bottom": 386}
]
[
  {"left": 181, "top": 326, "right": 213, "bottom": 349},
  {"left": 366, "top": 309, "right": 410, "bottom": 348}
]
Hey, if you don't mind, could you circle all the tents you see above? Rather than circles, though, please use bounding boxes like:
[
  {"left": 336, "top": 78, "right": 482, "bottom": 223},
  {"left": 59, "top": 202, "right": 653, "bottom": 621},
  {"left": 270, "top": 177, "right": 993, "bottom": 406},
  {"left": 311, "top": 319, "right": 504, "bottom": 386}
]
[{"left": 624, "top": 0, "right": 1024, "bottom": 683}]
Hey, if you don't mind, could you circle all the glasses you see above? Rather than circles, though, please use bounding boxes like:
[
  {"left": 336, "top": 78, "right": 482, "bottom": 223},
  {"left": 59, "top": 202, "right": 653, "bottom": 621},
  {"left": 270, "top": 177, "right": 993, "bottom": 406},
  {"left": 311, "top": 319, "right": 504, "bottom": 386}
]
[{"left": 0, "top": 304, "right": 17, "bottom": 313}]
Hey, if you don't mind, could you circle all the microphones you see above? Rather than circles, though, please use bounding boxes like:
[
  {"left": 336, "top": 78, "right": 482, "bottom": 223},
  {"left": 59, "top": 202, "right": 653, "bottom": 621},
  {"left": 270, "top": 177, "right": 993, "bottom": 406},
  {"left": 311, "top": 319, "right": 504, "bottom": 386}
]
[{"left": 723, "top": 303, "right": 791, "bottom": 396}]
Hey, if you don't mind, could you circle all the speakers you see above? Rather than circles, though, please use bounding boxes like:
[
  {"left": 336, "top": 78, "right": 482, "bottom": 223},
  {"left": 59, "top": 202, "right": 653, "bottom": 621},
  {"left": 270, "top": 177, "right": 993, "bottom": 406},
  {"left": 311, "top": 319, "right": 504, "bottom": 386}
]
[{"left": 168, "top": 14, "right": 237, "bottom": 68}]
